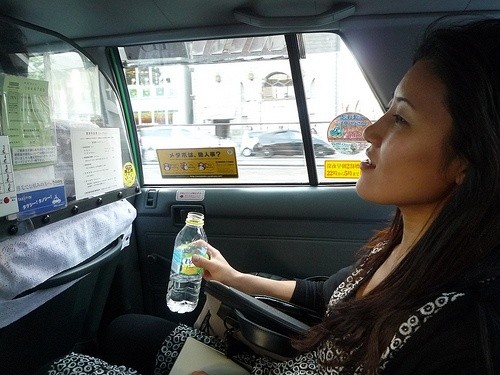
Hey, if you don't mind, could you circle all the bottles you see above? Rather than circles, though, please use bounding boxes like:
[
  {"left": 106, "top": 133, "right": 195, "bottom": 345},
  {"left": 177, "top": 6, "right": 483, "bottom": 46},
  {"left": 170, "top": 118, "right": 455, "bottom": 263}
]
[{"left": 166, "top": 212, "right": 208, "bottom": 314}]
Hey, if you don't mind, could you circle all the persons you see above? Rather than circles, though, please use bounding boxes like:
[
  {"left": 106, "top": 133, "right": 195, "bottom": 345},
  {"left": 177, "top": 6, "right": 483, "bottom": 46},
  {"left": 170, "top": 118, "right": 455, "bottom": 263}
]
[{"left": 36, "top": 17, "right": 500, "bottom": 375}]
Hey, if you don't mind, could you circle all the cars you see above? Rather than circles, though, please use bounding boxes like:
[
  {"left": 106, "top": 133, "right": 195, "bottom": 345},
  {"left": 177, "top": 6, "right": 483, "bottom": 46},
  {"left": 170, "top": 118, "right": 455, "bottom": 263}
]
[
  {"left": 257, "top": 130, "right": 335, "bottom": 158},
  {"left": 241, "top": 131, "right": 270, "bottom": 157}
]
